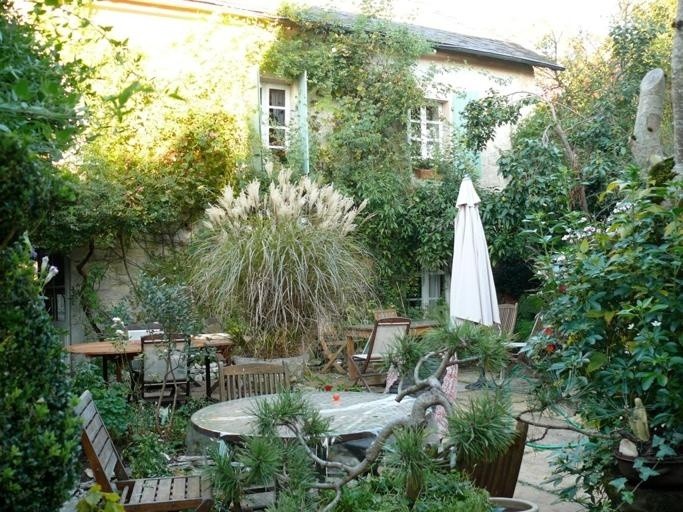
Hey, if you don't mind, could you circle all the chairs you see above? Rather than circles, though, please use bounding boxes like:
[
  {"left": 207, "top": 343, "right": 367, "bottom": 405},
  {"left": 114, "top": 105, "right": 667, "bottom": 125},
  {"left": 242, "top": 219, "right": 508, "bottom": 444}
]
[
  {"left": 71, "top": 390, "right": 213, "bottom": 512},
  {"left": 125, "top": 323, "right": 163, "bottom": 340},
  {"left": 127, "top": 333, "right": 191, "bottom": 408},
  {"left": 374, "top": 309, "right": 398, "bottom": 320},
  {"left": 349, "top": 317, "right": 411, "bottom": 392},
  {"left": 498, "top": 302, "right": 518, "bottom": 334},
  {"left": 457, "top": 421, "right": 528, "bottom": 496},
  {"left": 218, "top": 361, "right": 291, "bottom": 512}
]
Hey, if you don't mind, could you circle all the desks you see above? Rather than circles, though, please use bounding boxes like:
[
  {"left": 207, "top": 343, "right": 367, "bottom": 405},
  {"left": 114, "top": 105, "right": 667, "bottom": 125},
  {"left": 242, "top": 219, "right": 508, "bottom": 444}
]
[
  {"left": 343, "top": 321, "right": 440, "bottom": 381},
  {"left": 68, "top": 339, "right": 234, "bottom": 404},
  {"left": 190, "top": 391, "right": 433, "bottom": 484}
]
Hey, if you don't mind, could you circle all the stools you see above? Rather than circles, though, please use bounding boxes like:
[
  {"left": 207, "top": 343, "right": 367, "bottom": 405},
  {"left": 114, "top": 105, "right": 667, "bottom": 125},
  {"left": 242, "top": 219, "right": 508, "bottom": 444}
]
[{"left": 319, "top": 337, "right": 361, "bottom": 375}]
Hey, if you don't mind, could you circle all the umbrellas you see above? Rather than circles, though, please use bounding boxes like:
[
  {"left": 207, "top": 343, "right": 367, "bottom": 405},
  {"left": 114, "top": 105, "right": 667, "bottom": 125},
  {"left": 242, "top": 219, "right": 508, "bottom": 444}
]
[{"left": 448, "top": 173, "right": 502, "bottom": 377}]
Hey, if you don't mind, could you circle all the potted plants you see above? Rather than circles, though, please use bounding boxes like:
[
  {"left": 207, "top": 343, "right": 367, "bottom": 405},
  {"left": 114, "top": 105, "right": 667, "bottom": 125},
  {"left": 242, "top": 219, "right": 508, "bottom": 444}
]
[{"left": 517, "top": 154, "right": 683, "bottom": 512}]
